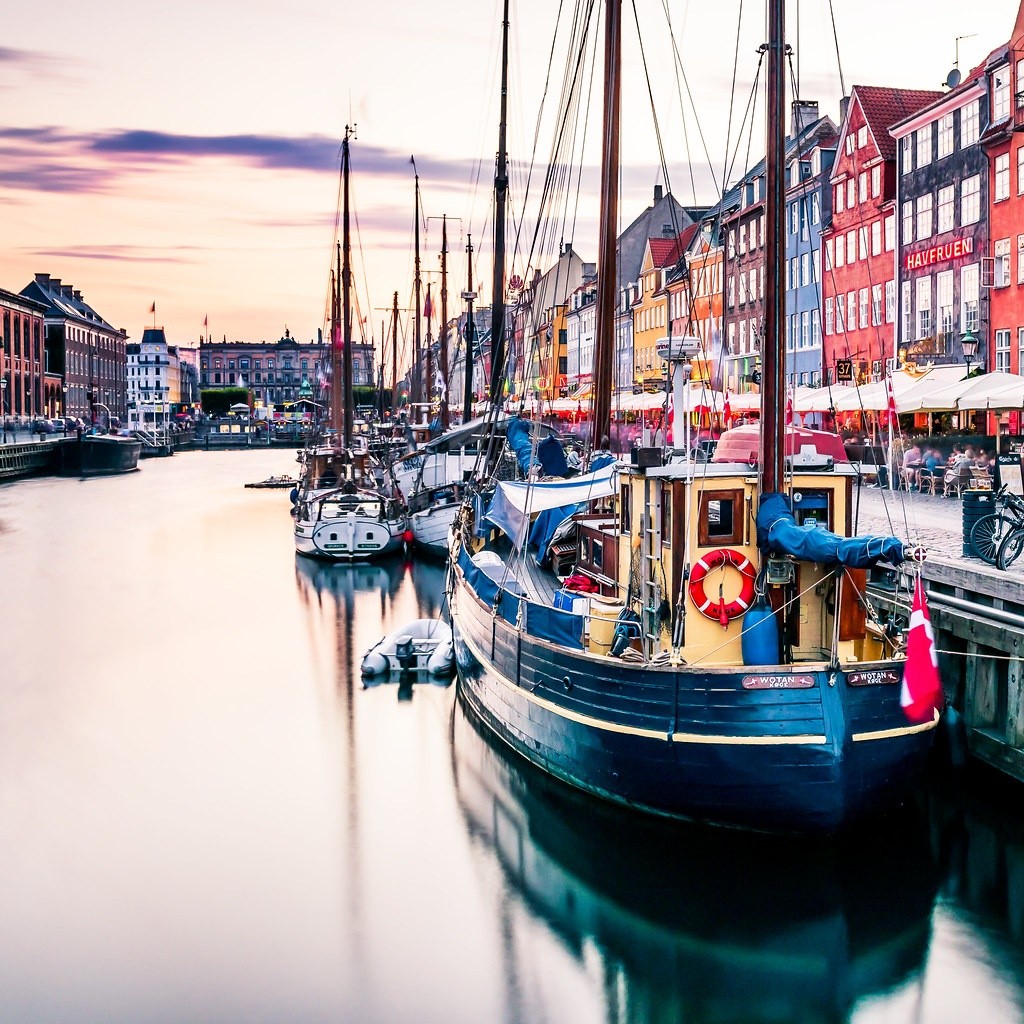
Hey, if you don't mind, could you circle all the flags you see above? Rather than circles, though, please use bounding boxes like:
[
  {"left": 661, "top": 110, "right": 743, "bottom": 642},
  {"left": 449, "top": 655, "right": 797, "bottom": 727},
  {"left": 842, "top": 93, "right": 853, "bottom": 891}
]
[
  {"left": 455, "top": 402, "right": 543, "bottom": 420},
  {"left": 721, "top": 386, "right": 732, "bottom": 423},
  {"left": 666, "top": 396, "right": 675, "bottom": 424},
  {"left": 574, "top": 399, "right": 585, "bottom": 418},
  {"left": 424, "top": 293, "right": 432, "bottom": 317},
  {"left": 902, "top": 577, "right": 942, "bottom": 723},
  {"left": 150, "top": 301, "right": 155, "bottom": 312},
  {"left": 883, "top": 379, "right": 899, "bottom": 427},
  {"left": 783, "top": 396, "right": 794, "bottom": 428},
  {"left": 202, "top": 315, "right": 207, "bottom": 325}
]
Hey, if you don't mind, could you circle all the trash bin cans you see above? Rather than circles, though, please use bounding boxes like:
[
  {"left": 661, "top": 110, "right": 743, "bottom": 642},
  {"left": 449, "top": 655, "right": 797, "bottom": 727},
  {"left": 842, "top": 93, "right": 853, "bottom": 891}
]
[{"left": 961, "top": 488, "right": 996, "bottom": 559}]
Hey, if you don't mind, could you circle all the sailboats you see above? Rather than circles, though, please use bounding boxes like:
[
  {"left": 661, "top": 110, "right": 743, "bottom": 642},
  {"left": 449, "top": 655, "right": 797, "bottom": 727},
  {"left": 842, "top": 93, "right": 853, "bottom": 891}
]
[{"left": 246, "top": 2, "right": 950, "bottom": 859}]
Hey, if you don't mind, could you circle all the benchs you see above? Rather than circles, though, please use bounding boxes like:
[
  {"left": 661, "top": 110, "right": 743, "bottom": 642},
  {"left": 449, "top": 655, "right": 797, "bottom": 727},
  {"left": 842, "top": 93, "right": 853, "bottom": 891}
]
[{"left": 552, "top": 544, "right": 576, "bottom": 575}]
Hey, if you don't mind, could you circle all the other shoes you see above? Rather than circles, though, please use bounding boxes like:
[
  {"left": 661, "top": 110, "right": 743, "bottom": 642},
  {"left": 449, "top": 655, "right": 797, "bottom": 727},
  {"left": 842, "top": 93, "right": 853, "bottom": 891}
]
[{"left": 941, "top": 494, "right": 947, "bottom": 498}]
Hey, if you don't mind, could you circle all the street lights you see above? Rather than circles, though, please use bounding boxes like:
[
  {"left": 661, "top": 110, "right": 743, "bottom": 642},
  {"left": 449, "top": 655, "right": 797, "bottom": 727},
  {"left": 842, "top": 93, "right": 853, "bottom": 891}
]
[
  {"left": 0, "top": 378, "right": 8, "bottom": 444},
  {"left": 62, "top": 383, "right": 69, "bottom": 437},
  {"left": 104, "top": 390, "right": 111, "bottom": 433},
  {"left": 26, "top": 388, "right": 31, "bottom": 439}
]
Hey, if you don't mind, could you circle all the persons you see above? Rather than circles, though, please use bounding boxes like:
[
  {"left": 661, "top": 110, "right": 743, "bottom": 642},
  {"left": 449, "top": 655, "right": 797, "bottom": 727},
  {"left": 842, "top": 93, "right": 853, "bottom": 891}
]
[
  {"left": 2, "top": 418, "right": 127, "bottom": 442},
  {"left": 157, "top": 408, "right": 416, "bottom": 436},
  {"left": 844, "top": 428, "right": 1000, "bottom": 498},
  {"left": 519, "top": 414, "right": 747, "bottom": 454},
  {"left": 567, "top": 447, "right": 584, "bottom": 465}
]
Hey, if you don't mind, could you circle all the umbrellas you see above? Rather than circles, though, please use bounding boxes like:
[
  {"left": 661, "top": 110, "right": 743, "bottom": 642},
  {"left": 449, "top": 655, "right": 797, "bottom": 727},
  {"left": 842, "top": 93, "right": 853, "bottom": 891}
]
[
  {"left": 440, "top": 396, "right": 538, "bottom": 417},
  {"left": 542, "top": 383, "right": 764, "bottom": 430},
  {"left": 788, "top": 366, "right": 1024, "bottom": 455}
]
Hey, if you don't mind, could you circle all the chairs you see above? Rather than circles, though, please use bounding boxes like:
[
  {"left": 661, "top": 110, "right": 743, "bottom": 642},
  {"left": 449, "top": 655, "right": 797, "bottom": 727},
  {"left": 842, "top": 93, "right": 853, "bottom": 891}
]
[{"left": 896, "top": 460, "right": 994, "bottom": 499}]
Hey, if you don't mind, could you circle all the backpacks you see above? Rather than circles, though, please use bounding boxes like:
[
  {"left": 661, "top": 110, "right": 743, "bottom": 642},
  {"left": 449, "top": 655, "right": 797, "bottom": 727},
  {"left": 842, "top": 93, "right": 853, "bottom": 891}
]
[{"left": 564, "top": 575, "right": 599, "bottom": 593}]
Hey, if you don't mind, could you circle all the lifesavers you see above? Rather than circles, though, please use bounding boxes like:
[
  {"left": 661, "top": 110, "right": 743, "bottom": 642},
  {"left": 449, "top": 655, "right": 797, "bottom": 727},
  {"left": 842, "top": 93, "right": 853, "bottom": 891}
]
[{"left": 690, "top": 548, "right": 759, "bottom": 624}]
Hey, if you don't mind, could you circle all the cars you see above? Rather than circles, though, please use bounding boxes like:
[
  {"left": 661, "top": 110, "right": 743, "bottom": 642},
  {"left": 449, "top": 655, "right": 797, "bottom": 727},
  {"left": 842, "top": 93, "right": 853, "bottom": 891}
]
[
  {"left": 106, "top": 416, "right": 121, "bottom": 428},
  {"left": 33, "top": 415, "right": 86, "bottom": 432}
]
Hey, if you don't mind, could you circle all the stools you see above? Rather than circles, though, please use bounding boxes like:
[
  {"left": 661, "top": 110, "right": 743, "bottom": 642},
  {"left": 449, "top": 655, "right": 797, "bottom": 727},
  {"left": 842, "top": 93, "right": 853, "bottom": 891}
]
[{"left": 412, "top": 638, "right": 443, "bottom": 651}]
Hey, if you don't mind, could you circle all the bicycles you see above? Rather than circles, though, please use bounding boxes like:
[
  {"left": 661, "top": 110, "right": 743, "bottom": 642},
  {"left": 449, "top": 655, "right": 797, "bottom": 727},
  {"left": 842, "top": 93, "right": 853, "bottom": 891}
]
[{"left": 970, "top": 483, "right": 1022, "bottom": 574}]
